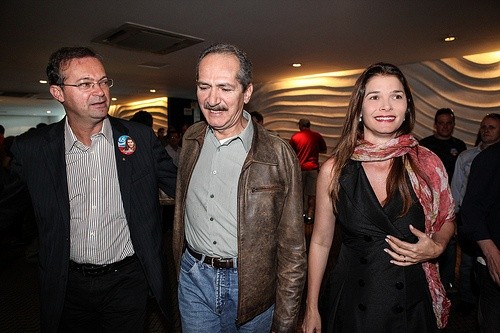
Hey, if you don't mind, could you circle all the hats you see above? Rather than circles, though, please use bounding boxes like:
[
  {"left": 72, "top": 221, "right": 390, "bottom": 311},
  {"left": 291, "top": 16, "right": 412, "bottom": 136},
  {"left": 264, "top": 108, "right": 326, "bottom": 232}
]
[{"left": 297, "top": 119, "right": 310, "bottom": 126}]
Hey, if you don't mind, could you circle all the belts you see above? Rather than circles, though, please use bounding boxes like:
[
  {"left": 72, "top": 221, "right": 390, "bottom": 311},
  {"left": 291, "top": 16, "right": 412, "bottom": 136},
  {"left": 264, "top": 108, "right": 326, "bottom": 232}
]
[
  {"left": 185, "top": 242, "right": 239, "bottom": 270},
  {"left": 71, "top": 257, "right": 135, "bottom": 277}
]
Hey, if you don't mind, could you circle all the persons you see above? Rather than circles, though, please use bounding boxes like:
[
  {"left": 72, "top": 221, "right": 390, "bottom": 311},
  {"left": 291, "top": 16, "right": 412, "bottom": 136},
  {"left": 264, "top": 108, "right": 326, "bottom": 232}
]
[
  {"left": 0, "top": 122, "right": 47, "bottom": 172},
  {"left": 461, "top": 139, "right": 499, "bottom": 332},
  {"left": 448, "top": 112, "right": 500, "bottom": 302},
  {"left": 125, "top": 138, "right": 136, "bottom": 150},
  {"left": 127, "top": 108, "right": 190, "bottom": 168},
  {"left": 288, "top": 117, "right": 328, "bottom": 223},
  {"left": 418, "top": 107, "right": 466, "bottom": 293},
  {"left": 300, "top": 60, "right": 456, "bottom": 333},
  {"left": 159, "top": 43, "right": 306, "bottom": 333},
  {"left": 0, "top": 45, "right": 178, "bottom": 332},
  {"left": 250, "top": 111, "right": 280, "bottom": 136}
]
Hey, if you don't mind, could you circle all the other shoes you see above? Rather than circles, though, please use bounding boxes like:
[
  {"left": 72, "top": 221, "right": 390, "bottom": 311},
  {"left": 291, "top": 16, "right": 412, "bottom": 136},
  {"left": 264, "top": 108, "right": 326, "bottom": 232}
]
[{"left": 304, "top": 214, "right": 313, "bottom": 224}]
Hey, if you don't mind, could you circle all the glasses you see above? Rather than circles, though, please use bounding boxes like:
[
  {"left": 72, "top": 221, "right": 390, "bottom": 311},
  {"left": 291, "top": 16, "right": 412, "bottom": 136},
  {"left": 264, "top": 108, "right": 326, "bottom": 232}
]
[{"left": 56, "top": 78, "right": 113, "bottom": 92}]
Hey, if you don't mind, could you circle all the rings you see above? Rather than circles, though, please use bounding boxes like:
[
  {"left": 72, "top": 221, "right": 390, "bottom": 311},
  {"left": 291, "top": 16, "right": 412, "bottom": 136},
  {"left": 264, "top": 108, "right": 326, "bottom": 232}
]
[{"left": 404, "top": 255, "right": 406, "bottom": 261}]
